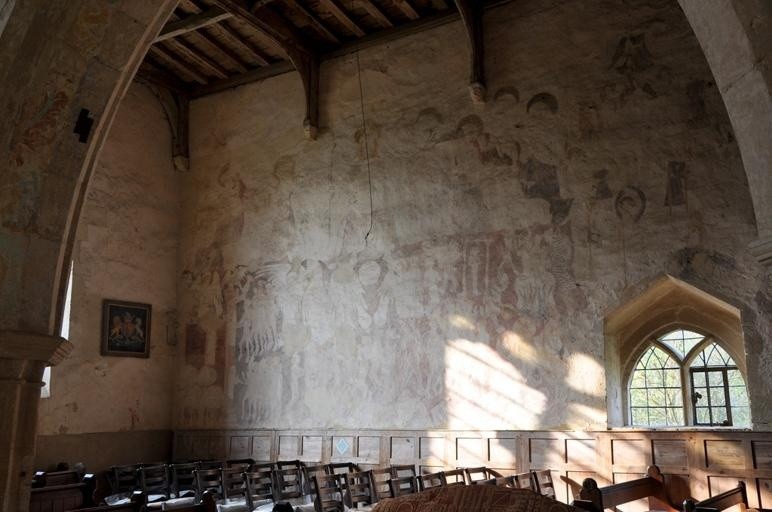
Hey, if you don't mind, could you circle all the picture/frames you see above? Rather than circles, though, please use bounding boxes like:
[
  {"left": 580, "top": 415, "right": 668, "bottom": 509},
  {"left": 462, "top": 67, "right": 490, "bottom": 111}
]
[{"left": 99, "top": 297, "right": 154, "bottom": 361}]
